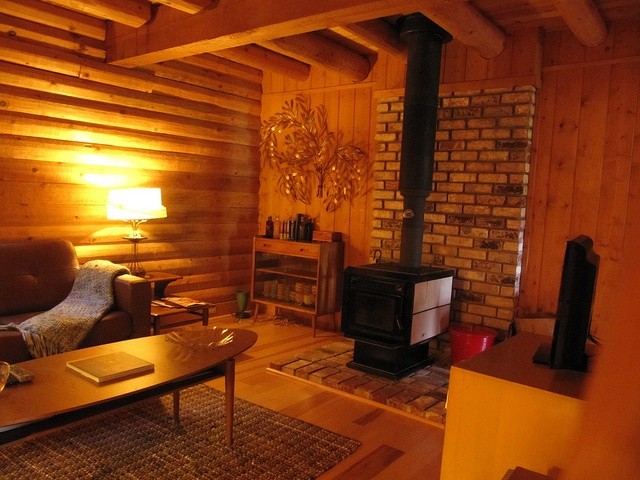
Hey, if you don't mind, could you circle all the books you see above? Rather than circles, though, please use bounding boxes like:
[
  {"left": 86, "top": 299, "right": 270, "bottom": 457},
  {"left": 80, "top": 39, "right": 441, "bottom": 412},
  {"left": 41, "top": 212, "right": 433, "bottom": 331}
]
[{"left": 66, "top": 352, "right": 154, "bottom": 383}]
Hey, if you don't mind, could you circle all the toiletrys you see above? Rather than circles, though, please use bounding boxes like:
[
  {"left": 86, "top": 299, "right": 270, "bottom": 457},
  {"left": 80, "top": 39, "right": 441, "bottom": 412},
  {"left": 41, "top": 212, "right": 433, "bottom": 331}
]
[{"left": 280, "top": 219, "right": 294, "bottom": 240}]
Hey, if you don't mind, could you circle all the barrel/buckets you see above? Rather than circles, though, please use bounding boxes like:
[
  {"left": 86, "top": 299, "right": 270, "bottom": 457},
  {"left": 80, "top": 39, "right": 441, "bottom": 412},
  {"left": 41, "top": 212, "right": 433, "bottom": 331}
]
[{"left": 450, "top": 326, "right": 497, "bottom": 368}]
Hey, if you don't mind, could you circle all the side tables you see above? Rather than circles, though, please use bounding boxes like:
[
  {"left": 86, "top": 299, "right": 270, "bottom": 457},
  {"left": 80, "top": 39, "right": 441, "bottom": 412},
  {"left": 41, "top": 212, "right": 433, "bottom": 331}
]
[
  {"left": 152, "top": 301, "right": 215, "bottom": 333},
  {"left": 122, "top": 269, "right": 183, "bottom": 298}
]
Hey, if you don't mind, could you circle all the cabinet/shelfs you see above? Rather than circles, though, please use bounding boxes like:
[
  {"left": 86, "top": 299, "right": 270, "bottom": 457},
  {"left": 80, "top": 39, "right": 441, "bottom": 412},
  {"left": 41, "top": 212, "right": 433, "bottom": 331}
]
[
  {"left": 438, "top": 331, "right": 589, "bottom": 479},
  {"left": 249, "top": 230, "right": 343, "bottom": 339}
]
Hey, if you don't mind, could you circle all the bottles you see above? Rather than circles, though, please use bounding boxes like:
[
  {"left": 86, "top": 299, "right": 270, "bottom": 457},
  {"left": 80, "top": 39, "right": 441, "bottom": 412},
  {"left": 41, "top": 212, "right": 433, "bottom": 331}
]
[{"left": 265, "top": 213, "right": 315, "bottom": 242}]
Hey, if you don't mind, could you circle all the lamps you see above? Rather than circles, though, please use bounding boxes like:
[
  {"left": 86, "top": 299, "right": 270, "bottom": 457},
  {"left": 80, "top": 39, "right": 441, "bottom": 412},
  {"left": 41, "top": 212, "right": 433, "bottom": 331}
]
[{"left": 106, "top": 186, "right": 166, "bottom": 278}]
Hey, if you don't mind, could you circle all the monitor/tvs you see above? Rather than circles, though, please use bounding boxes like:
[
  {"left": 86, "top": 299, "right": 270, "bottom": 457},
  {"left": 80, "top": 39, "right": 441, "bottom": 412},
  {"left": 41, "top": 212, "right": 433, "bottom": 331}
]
[{"left": 531, "top": 234, "right": 600, "bottom": 373}]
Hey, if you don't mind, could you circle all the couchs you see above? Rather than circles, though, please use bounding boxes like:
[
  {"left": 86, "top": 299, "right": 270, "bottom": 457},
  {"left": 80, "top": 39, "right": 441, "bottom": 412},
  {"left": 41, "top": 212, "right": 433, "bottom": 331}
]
[{"left": 0, "top": 239, "right": 151, "bottom": 364}]
